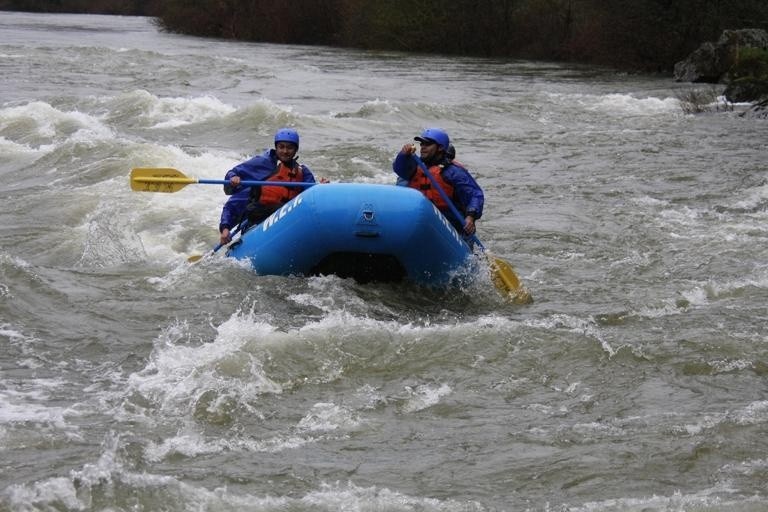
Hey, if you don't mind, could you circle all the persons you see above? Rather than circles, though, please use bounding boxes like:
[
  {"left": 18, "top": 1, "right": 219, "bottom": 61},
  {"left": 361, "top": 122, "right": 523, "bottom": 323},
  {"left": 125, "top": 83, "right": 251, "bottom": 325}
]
[
  {"left": 223, "top": 129, "right": 330, "bottom": 224},
  {"left": 219, "top": 186, "right": 255, "bottom": 246},
  {"left": 393, "top": 129, "right": 484, "bottom": 235}
]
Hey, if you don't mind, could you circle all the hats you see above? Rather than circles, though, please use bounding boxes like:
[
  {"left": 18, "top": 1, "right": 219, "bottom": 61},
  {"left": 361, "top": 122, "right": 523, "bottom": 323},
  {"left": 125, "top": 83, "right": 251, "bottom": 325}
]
[{"left": 414, "top": 136, "right": 435, "bottom": 143}]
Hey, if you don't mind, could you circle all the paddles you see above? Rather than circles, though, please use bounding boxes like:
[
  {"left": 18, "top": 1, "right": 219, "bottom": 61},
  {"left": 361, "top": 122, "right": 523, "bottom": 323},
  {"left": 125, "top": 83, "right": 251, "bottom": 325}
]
[
  {"left": 128, "top": 167, "right": 319, "bottom": 193},
  {"left": 410, "top": 145, "right": 519, "bottom": 293},
  {"left": 187, "top": 219, "right": 250, "bottom": 263}
]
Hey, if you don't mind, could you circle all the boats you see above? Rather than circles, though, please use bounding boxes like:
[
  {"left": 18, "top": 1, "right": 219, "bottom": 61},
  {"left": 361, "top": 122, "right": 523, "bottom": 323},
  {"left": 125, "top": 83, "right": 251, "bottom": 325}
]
[{"left": 218, "top": 182, "right": 478, "bottom": 290}]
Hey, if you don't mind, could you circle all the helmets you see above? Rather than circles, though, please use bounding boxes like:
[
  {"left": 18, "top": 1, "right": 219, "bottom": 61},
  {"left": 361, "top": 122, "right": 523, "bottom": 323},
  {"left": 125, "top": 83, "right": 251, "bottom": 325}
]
[
  {"left": 421, "top": 128, "right": 450, "bottom": 153},
  {"left": 274, "top": 129, "right": 299, "bottom": 153}
]
